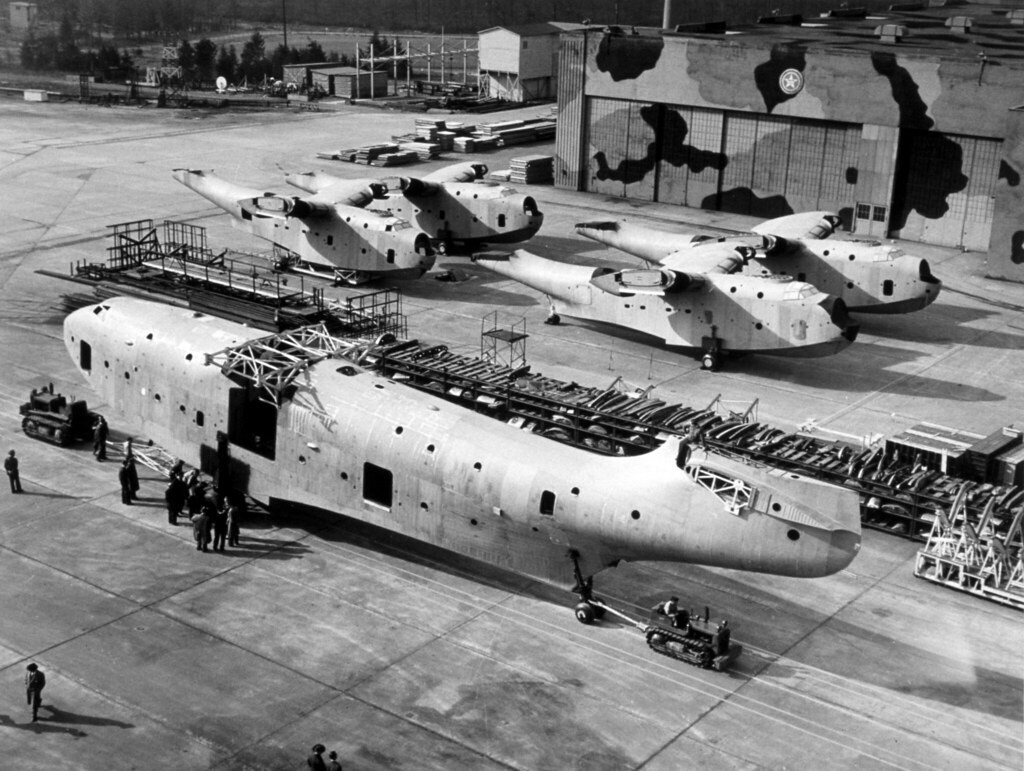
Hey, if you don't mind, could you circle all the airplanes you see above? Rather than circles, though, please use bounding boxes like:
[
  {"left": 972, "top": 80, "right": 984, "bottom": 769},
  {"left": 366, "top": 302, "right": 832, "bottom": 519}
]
[
  {"left": 171, "top": 162, "right": 545, "bottom": 287},
  {"left": 60, "top": 294, "right": 862, "bottom": 627},
  {"left": 473, "top": 212, "right": 939, "bottom": 371}
]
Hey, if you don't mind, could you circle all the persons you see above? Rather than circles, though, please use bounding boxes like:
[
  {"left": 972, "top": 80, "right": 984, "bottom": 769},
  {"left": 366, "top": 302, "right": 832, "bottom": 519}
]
[
  {"left": 306, "top": 744, "right": 327, "bottom": 771},
  {"left": 25, "top": 664, "right": 46, "bottom": 723},
  {"left": 119, "top": 436, "right": 139, "bottom": 504},
  {"left": 326, "top": 751, "right": 342, "bottom": 771},
  {"left": 665, "top": 596, "right": 679, "bottom": 626},
  {"left": 163, "top": 460, "right": 241, "bottom": 553},
  {"left": 4, "top": 450, "right": 23, "bottom": 493},
  {"left": 94, "top": 416, "right": 110, "bottom": 461}
]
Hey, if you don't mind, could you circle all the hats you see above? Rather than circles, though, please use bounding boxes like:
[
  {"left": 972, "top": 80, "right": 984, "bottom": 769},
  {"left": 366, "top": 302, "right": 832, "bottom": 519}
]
[
  {"left": 200, "top": 506, "right": 208, "bottom": 513},
  {"left": 312, "top": 743, "right": 326, "bottom": 754},
  {"left": 26, "top": 663, "right": 38, "bottom": 672}
]
